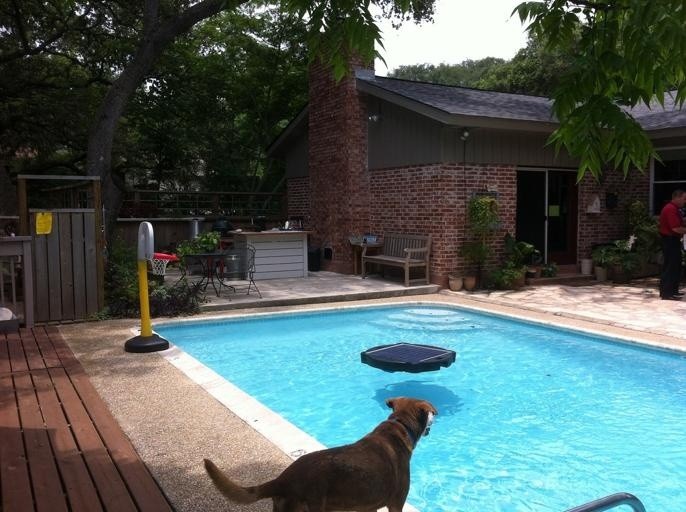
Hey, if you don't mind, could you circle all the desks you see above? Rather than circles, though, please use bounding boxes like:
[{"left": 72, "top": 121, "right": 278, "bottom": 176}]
[{"left": 229, "top": 228, "right": 315, "bottom": 281}]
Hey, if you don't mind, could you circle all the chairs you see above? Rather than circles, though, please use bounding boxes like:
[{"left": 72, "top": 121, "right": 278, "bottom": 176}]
[
  {"left": 173, "top": 255, "right": 205, "bottom": 295},
  {"left": 217, "top": 242, "right": 262, "bottom": 298}
]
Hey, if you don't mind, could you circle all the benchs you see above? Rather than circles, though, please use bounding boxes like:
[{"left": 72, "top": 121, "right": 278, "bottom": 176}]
[{"left": 360, "top": 231, "right": 433, "bottom": 287}]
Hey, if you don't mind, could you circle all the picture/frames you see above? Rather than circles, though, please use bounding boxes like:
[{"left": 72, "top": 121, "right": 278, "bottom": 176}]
[{"left": 284, "top": 217, "right": 304, "bottom": 231}]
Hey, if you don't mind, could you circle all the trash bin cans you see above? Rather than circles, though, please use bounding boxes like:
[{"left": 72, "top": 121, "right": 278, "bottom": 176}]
[
  {"left": 308, "top": 247, "right": 320, "bottom": 271},
  {"left": 225, "top": 254, "right": 242, "bottom": 279}
]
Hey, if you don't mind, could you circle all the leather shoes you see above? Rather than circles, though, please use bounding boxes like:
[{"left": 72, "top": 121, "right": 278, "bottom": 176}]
[
  {"left": 663, "top": 296, "right": 681, "bottom": 300},
  {"left": 673, "top": 292, "right": 683, "bottom": 295}
]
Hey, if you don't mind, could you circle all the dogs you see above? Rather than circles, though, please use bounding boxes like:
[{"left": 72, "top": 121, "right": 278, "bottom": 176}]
[{"left": 203, "top": 396, "right": 439, "bottom": 512}]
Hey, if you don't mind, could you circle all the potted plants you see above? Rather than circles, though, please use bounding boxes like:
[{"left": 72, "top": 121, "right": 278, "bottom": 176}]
[
  {"left": 581, "top": 238, "right": 614, "bottom": 283},
  {"left": 448, "top": 194, "right": 560, "bottom": 291}
]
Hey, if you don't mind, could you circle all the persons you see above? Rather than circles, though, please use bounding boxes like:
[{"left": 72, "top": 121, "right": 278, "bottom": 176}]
[{"left": 657, "top": 190, "right": 686, "bottom": 301}]
